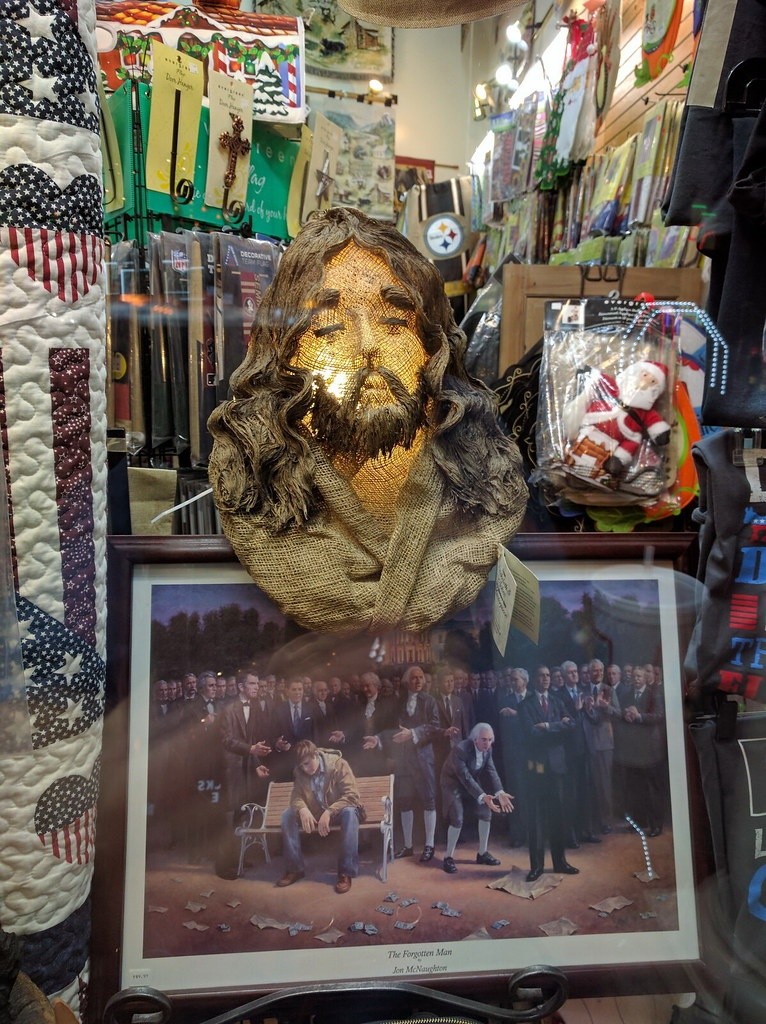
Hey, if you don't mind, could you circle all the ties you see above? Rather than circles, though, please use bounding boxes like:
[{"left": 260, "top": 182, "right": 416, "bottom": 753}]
[
  {"left": 518, "top": 695, "right": 523, "bottom": 703},
  {"left": 635, "top": 691, "right": 641, "bottom": 702},
  {"left": 445, "top": 697, "right": 452, "bottom": 723},
  {"left": 594, "top": 686, "right": 598, "bottom": 702},
  {"left": 541, "top": 695, "right": 548, "bottom": 716},
  {"left": 571, "top": 688, "right": 577, "bottom": 702},
  {"left": 293, "top": 705, "right": 300, "bottom": 736}
]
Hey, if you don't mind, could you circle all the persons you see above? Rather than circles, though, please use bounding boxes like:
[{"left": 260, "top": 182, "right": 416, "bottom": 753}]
[
  {"left": 209, "top": 207, "right": 528, "bottom": 635},
  {"left": 152, "top": 660, "right": 668, "bottom": 893}
]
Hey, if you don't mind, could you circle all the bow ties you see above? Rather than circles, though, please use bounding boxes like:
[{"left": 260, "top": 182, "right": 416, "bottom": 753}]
[
  {"left": 206, "top": 699, "right": 215, "bottom": 707},
  {"left": 243, "top": 702, "right": 254, "bottom": 709},
  {"left": 161, "top": 698, "right": 171, "bottom": 706}
]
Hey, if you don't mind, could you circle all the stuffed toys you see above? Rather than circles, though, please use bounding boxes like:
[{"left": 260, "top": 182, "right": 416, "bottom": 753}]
[{"left": 564, "top": 360, "right": 672, "bottom": 490}]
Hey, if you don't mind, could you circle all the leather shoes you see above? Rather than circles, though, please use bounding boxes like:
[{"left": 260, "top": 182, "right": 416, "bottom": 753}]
[
  {"left": 390, "top": 846, "right": 413, "bottom": 858},
  {"left": 336, "top": 871, "right": 351, "bottom": 893},
  {"left": 527, "top": 867, "right": 544, "bottom": 881},
  {"left": 555, "top": 864, "right": 579, "bottom": 874},
  {"left": 510, "top": 821, "right": 662, "bottom": 849},
  {"left": 419, "top": 846, "right": 435, "bottom": 862},
  {"left": 277, "top": 869, "right": 304, "bottom": 886},
  {"left": 444, "top": 857, "right": 458, "bottom": 873},
  {"left": 477, "top": 852, "right": 500, "bottom": 865}
]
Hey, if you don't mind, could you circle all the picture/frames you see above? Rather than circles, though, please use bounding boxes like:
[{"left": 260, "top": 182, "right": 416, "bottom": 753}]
[{"left": 86, "top": 532, "right": 715, "bottom": 1017}]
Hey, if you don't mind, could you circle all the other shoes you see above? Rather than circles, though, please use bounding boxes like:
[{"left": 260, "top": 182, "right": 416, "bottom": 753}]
[
  {"left": 232, "top": 860, "right": 252, "bottom": 868},
  {"left": 218, "top": 870, "right": 238, "bottom": 880}
]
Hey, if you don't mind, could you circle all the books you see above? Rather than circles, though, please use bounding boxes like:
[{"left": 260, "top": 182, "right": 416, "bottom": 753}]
[{"left": 107, "top": 231, "right": 284, "bottom": 535}]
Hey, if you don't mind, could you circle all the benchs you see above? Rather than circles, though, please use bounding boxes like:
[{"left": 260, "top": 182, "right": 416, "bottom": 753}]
[{"left": 237, "top": 775, "right": 396, "bottom": 884}]
